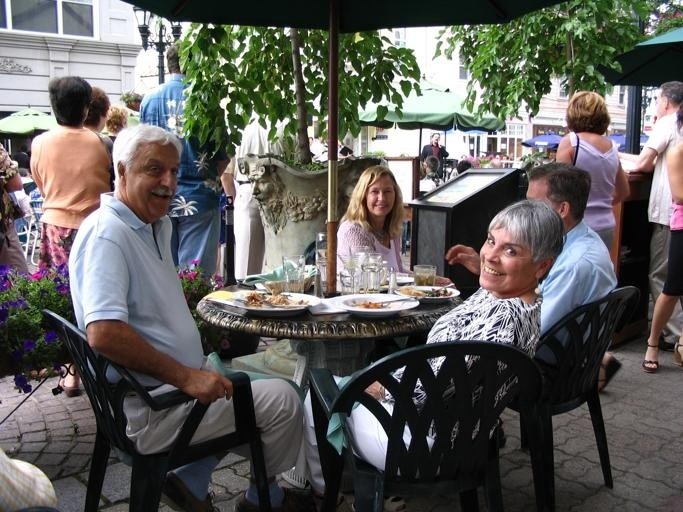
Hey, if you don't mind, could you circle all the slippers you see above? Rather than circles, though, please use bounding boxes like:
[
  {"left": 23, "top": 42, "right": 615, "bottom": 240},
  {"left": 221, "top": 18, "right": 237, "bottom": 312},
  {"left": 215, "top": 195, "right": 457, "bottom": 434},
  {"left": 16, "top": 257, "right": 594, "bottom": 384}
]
[{"left": 59, "top": 379, "right": 83, "bottom": 398}]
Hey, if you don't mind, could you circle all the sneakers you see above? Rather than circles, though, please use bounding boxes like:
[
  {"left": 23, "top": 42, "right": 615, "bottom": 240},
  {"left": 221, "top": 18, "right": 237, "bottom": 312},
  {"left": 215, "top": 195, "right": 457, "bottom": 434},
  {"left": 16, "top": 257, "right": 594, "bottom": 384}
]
[
  {"left": 374, "top": 493, "right": 406, "bottom": 512},
  {"left": 318, "top": 490, "right": 355, "bottom": 511},
  {"left": 161, "top": 472, "right": 220, "bottom": 512},
  {"left": 234, "top": 486, "right": 318, "bottom": 511}
]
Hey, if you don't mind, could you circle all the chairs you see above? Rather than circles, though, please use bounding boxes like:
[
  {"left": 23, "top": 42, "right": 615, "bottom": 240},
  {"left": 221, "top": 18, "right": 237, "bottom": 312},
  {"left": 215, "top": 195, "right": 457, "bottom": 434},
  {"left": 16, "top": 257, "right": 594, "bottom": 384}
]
[
  {"left": 505, "top": 286, "right": 640, "bottom": 512},
  {"left": 298, "top": 240, "right": 316, "bottom": 267},
  {"left": 308, "top": 340, "right": 544, "bottom": 512},
  {"left": 40, "top": 308, "right": 271, "bottom": 512}
]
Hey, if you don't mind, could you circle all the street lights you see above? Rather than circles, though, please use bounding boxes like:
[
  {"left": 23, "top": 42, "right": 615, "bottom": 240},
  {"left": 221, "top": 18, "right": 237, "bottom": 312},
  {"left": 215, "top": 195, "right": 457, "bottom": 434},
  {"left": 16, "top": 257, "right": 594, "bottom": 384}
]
[{"left": 132, "top": 2, "right": 184, "bottom": 92}]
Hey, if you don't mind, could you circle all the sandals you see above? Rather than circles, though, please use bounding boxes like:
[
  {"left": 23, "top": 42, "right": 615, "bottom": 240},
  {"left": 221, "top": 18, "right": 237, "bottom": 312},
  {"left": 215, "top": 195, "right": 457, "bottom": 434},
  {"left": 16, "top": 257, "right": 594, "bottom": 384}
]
[
  {"left": 642, "top": 345, "right": 660, "bottom": 373},
  {"left": 673, "top": 343, "right": 682, "bottom": 366},
  {"left": 599, "top": 356, "right": 622, "bottom": 391}
]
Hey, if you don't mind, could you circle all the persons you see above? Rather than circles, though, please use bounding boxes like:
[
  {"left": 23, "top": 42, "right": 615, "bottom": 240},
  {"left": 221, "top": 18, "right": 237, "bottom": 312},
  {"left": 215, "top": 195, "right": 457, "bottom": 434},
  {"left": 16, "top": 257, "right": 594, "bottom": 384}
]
[
  {"left": 336, "top": 165, "right": 453, "bottom": 296},
  {"left": 635, "top": 80, "right": 683, "bottom": 366},
  {"left": 68, "top": 123, "right": 322, "bottom": 512},
  {"left": 281, "top": 198, "right": 566, "bottom": 500},
  {"left": 556, "top": 90, "right": 631, "bottom": 393},
  {"left": 642, "top": 100, "right": 683, "bottom": 374},
  {"left": 0, "top": 44, "right": 329, "bottom": 397},
  {"left": 445, "top": 163, "right": 619, "bottom": 405},
  {"left": 418, "top": 133, "right": 534, "bottom": 199}
]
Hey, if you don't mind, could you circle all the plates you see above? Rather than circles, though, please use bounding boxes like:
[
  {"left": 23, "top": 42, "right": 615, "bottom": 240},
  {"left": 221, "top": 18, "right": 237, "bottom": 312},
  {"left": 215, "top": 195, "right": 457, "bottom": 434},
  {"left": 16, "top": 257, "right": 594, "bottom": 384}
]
[
  {"left": 329, "top": 293, "right": 421, "bottom": 320},
  {"left": 387, "top": 276, "right": 414, "bottom": 285},
  {"left": 394, "top": 285, "right": 461, "bottom": 304},
  {"left": 231, "top": 290, "right": 322, "bottom": 318}
]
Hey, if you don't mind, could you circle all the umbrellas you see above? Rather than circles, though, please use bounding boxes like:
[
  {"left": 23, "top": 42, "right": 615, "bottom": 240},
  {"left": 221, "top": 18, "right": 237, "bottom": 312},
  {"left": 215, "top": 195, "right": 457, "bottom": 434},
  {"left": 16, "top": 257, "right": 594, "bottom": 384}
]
[
  {"left": 358, "top": 72, "right": 505, "bottom": 157},
  {"left": 125, "top": 0, "right": 573, "bottom": 298},
  {"left": 520, "top": 133, "right": 564, "bottom": 149},
  {"left": 603, "top": 26, "right": 683, "bottom": 86},
  {"left": 602, "top": 133, "right": 628, "bottom": 151}
]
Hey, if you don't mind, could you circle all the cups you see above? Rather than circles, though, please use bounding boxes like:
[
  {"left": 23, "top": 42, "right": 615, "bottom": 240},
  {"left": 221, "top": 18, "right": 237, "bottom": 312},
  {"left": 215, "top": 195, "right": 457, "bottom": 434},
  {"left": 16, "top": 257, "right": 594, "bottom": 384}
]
[
  {"left": 282, "top": 254, "right": 306, "bottom": 293},
  {"left": 314, "top": 232, "right": 389, "bottom": 296},
  {"left": 413, "top": 264, "right": 438, "bottom": 285}
]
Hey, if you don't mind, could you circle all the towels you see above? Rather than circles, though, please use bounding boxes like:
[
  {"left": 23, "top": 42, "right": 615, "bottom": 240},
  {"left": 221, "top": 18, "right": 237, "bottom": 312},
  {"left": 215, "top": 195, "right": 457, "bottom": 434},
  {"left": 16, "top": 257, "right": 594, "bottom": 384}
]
[
  {"left": 241, "top": 259, "right": 316, "bottom": 287},
  {"left": 206, "top": 352, "right": 272, "bottom": 382},
  {"left": 326, "top": 376, "right": 360, "bottom": 457}
]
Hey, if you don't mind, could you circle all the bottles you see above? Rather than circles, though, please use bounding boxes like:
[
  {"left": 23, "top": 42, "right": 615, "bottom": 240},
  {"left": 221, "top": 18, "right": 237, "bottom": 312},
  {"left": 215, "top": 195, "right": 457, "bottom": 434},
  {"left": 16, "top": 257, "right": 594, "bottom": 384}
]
[{"left": 387, "top": 266, "right": 399, "bottom": 293}]
